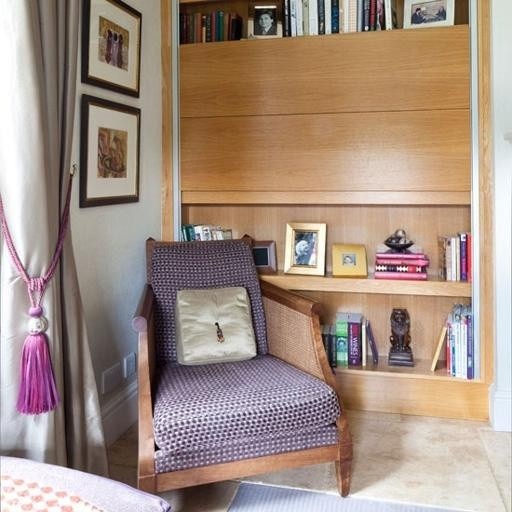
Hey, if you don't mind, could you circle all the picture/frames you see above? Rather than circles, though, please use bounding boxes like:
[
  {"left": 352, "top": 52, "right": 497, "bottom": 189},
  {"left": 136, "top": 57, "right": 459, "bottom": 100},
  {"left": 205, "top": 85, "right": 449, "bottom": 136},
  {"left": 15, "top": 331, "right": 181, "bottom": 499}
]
[
  {"left": 247, "top": 1, "right": 288, "bottom": 42},
  {"left": 78, "top": 92, "right": 142, "bottom": 210},
  {"left": 81, "top": 0, "right": 144, "bottom": 99},
  {"left": 285, "top": 221, "right": 327, "bottom": 277},
  {"left": 330, "top": 242, "right": 369, "bottom": 279},
  {"left": 402, "top": 0, "right": 456, "bottom": 30}
]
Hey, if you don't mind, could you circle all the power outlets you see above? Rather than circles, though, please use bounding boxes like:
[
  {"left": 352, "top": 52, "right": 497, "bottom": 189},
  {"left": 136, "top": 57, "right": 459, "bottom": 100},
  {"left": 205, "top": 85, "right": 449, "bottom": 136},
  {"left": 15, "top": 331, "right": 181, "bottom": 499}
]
[
  {"left": 103, "top": 361, "right": 124, "bottom": 393},
  {"left": 123, "top": 351, "right": 136, "bottom": 378}
]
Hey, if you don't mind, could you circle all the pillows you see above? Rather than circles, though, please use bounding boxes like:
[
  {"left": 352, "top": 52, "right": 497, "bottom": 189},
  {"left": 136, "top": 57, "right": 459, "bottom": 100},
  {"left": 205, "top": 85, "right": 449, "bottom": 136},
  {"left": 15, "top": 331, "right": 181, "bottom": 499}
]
[{"left": 173, "top": 287, "right": 259, "bottom": 367}]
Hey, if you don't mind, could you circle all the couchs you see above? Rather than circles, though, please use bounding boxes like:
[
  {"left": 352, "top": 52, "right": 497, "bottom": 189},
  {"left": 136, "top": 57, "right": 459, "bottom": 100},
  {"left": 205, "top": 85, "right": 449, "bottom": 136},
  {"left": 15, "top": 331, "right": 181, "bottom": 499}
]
[{"left": 130, "top": 233, "right": 352, "bottom": 498}]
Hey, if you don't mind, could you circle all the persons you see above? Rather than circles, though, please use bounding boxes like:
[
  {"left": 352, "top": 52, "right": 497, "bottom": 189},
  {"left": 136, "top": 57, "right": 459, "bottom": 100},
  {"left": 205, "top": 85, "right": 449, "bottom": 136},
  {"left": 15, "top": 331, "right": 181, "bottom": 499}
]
[
  {"left": 294, "top": 240, "right": 315, "bottom": 266},
  {"left": 105, "top": 29, "right": 124, "bottom": 70}
]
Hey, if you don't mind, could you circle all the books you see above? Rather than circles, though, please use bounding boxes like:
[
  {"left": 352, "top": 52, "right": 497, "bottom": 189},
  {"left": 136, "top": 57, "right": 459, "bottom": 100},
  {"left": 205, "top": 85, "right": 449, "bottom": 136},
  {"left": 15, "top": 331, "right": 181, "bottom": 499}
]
[
  {"left": 319, "top": 231, "right": 475, "bottom": 380},
  {"left": 181, "top": 223, "right": 235, "bottom": 241},
  {"left": 180, "top": 0, "right": 457, "bottom": 45}
]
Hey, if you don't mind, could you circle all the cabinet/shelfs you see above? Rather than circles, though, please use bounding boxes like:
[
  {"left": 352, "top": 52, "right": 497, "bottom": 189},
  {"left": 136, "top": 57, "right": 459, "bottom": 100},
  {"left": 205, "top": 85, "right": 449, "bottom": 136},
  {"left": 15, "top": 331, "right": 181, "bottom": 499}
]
[{"left": 160, "top": 0, "right": 496, "bottom": 430}]
